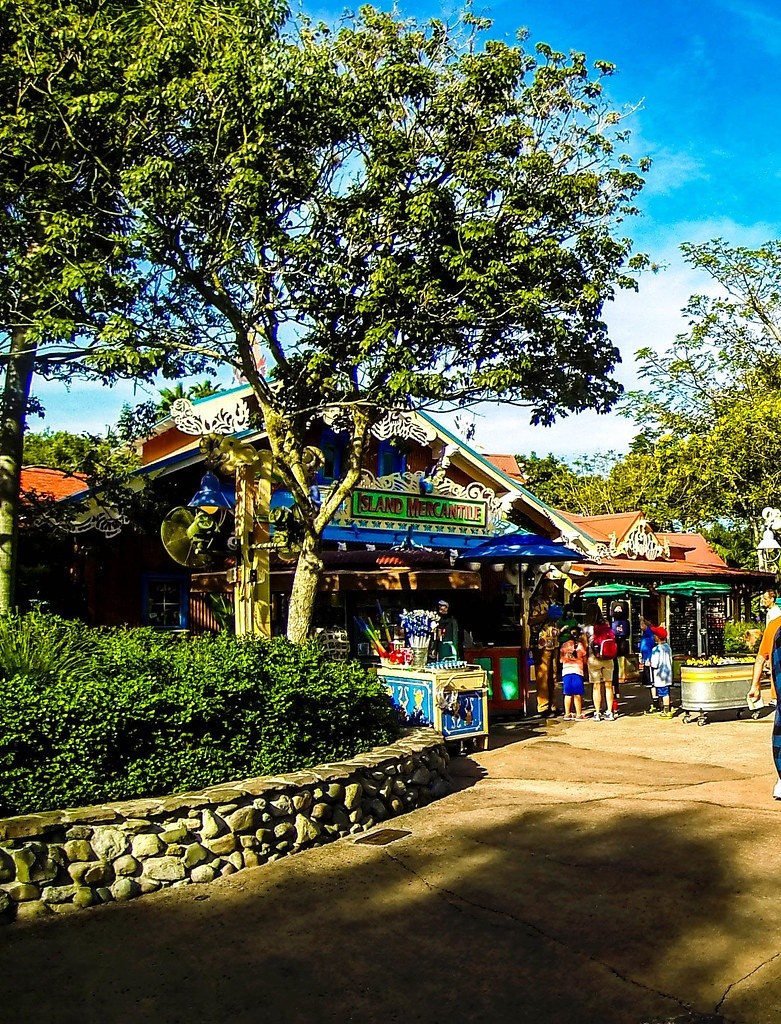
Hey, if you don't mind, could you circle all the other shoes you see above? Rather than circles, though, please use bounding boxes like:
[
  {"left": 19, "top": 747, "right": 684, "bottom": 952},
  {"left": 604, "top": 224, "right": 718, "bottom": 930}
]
[
  {"left": 593, "top": 712, "right": 602, "bottom": 721},
  {"left": 616, "top": 694, "right": 620, "bottom": 698},
  {"left": 563, "top": 712, "right": 576, "bottom": 720},
  {"left": 575, "top": 713, "right": 591, "bottom": 721},
  {"left": 602, "top": 711, "right": 614, "bottom": 721},
  {"left": 612, "top": 709, "right": 619, "bottom": 718},
  {"left": 773, "top": 777, "right": 781, "bottom": 798}
]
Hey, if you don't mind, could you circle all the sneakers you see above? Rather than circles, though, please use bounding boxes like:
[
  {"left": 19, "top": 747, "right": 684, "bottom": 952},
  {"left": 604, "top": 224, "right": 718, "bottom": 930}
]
[
  {"left": 643, "top": 705, "right": 662, "bottom": 716},
  {"left": 657, "top": 712, "right": 672, "bottom": 719}
]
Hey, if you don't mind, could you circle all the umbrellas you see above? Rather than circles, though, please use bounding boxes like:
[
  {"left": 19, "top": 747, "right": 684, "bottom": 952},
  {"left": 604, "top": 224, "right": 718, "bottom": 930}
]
[{"left": 458, "top": 529, "right": 587, "bottom": 716}]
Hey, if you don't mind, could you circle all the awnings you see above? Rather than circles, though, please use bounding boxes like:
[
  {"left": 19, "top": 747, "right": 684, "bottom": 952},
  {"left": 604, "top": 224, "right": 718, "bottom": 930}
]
[
  {"left": 582, "top": 583, "right": 651, "bottom": 599},
  {"left": 654, "top": 580, "right": 731, "bottom": 596}
]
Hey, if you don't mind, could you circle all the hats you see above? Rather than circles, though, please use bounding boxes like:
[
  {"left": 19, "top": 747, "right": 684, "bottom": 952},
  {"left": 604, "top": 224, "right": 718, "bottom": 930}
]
[
  {"left": 650, "top": 626, "right": 668, "bottom": 639},
  {"left": 438, "top": 600, "right": 449, "bottom": 608}
]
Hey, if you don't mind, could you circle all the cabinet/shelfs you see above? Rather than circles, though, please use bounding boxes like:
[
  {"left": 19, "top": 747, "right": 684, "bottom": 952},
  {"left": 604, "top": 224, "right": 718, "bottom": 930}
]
[{"left": 462, "top": 646, "right": 530, "bottom": 712}]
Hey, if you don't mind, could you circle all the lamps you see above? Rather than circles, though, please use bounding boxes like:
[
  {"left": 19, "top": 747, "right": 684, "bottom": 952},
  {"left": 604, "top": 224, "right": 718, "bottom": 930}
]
[{"left": 468, "top": 560, "right": 572, "bottom": 576}]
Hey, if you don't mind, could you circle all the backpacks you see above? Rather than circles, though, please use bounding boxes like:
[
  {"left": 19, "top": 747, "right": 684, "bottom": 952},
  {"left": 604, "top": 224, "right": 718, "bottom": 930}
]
[
  {"left": 590, "top": 619, "right": 617, "bottom": 660},
  {"left": 537, "top": 621, "right": 560, "bottom": 650},
  {"left": 611, "top": 619, "right": 628, "bottom": 635}
]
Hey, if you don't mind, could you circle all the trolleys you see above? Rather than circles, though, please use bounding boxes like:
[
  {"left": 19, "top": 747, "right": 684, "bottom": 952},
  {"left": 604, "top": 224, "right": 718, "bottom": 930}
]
[{"left": 681, "top": 661, "right": 761, "bottom": 726}]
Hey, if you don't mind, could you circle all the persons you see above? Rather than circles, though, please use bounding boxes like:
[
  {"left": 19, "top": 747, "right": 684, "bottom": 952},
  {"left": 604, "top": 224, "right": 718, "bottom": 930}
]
[
  {"left": 486, "top": 594, "right": 518, "bottom": 628},
  {"left": 436, "top": 601, "right": 459, "bottom": 659},
  {"left": 637, "top": 615, "right": 674, "bottom": 719},
  {"left": 582, "top": 604, "right": 616, "bottom": 721},
  {"left": 748, "top": 584, "right": 781, "bottom": 801},
  {"left": 558, "top": 627, "right": 589, "bottom": 721}
]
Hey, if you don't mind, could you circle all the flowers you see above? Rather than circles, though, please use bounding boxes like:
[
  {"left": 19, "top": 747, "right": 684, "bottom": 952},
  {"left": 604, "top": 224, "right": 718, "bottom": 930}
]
[{"left": 684, "top": 655, "right": 757, "bottom": 666}]
